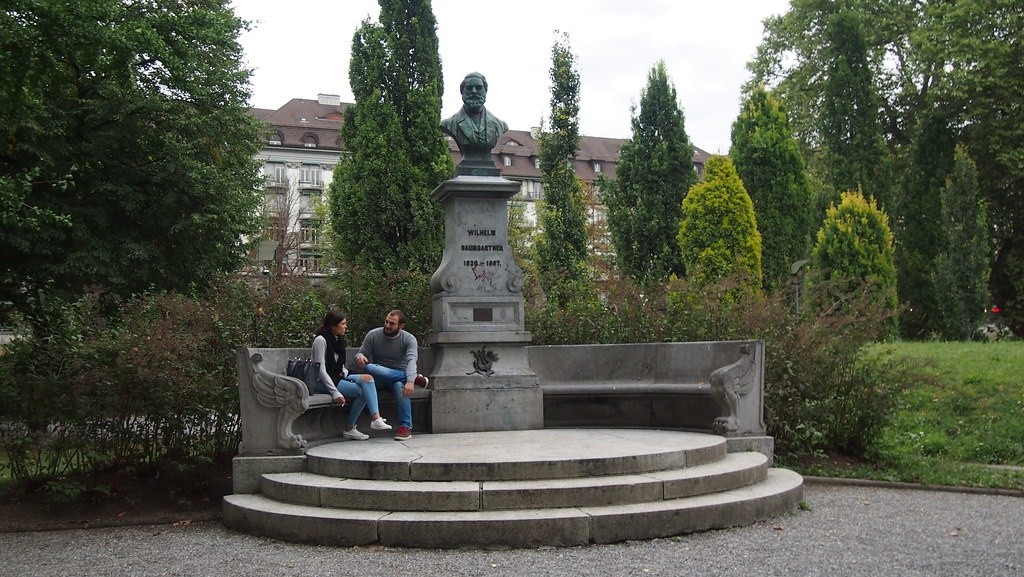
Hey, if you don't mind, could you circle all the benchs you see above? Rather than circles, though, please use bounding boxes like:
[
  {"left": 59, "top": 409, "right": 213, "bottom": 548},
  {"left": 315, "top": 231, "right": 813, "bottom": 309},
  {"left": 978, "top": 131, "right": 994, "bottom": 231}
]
[{"left": 236, "top": 340, "right": 765, "bottom": 457}]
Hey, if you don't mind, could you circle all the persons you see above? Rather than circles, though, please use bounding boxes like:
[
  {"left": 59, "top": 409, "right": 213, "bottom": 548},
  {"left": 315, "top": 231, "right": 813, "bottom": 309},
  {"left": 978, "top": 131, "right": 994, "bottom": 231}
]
[
  {"left": 355, "top": 310, "right": 429, "bottom": 439},
  {"left": 439, "top": 72, "right": 508, "bottom": 150},
  {"left": 311, "top": 311, "right": 393, "bottom": 440}
]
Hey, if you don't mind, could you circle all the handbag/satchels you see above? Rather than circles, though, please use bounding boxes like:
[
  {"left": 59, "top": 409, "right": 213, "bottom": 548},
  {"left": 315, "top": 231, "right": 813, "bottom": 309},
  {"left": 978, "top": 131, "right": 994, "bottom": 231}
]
[{"left": 286, "top": 356, "right": 321, "bottom": 396}]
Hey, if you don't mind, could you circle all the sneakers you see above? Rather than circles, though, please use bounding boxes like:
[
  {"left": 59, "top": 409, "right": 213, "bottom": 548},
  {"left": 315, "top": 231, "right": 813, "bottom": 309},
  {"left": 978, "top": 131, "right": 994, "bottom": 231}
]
[
  {"left": 393, "top": 425, "right": 412, "bottom": 440},
  {"left": 370, "top": 417, "right": 392, "bottom": 431},
  {"left": 414, "top": 373, "right": 429, "bottom": 389},
  {"left": 342, "top": 424, "right": 370, "bottom": 441}
]
